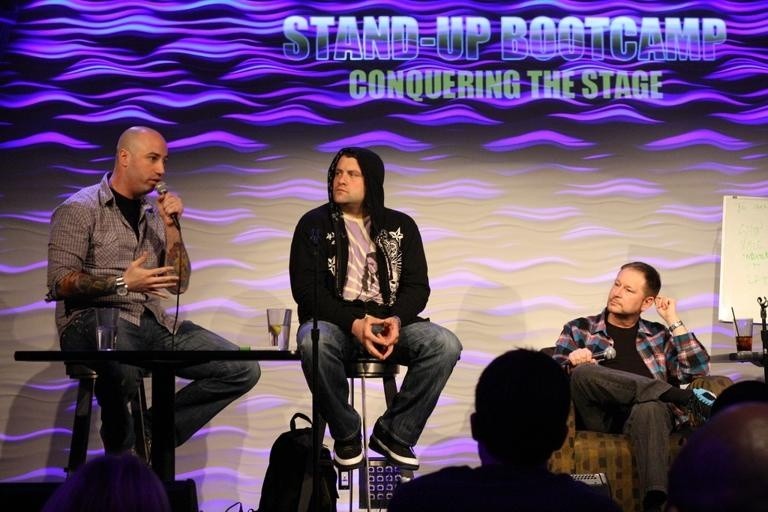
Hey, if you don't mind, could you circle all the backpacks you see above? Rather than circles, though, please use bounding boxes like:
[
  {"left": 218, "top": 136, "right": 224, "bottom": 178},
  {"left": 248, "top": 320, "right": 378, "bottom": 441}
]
[{"left": 258, "top": 414, "right": 339, "bottom": 512}]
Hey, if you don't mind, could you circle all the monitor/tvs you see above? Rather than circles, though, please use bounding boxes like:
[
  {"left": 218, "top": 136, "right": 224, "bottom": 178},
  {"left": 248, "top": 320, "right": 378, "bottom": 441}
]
[{"left": 559, "top": 346, "right": 617, "bottom": 368}]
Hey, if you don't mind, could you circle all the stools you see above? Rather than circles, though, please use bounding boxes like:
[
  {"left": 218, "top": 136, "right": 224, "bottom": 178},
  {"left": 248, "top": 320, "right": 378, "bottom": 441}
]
[
  {"left": 304, "top": 352, "right": 418, "bottom": 512},
  {"left": 65, "top": 362, "right": 152, "bottom": 479}
]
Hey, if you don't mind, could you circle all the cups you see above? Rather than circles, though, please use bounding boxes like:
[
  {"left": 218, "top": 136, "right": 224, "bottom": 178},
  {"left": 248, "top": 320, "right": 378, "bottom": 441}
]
[
  {"left": 266, "top": 307, "right": 293, "bottom": 350},
  {"left": 94, "top": 308, "right": 119, "bottom": 351},
  {"left": 733, "top": 318, "right": 753, "bottom": 351}
]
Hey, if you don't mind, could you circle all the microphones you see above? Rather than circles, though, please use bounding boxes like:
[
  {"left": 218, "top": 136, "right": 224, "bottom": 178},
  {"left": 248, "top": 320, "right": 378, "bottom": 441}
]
[{"left": 152, "top": 179, "right": 185, "bottom": 235}]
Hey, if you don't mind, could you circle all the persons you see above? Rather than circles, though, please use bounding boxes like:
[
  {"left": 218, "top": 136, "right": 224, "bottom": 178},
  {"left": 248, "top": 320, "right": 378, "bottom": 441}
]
[
  {"left": 666, "top": 404, "right": 768, "bottom": 512},
  {"left": 289, "top": 147, "right": 462, "bottom": 468},
  {"left": 46, "top": 127, "right": 262, "bottom": 471},
  {"left": 552, "top": 263, "right": 720, "bottom": 511},
  {"left": 387, "top": 349, "right": 621, "bottom": 512}
]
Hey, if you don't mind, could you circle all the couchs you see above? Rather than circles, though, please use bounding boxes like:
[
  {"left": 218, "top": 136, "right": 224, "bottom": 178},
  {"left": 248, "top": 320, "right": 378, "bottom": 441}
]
[{"left": 542, "top": 347, "right": 735, "bottom": 505}]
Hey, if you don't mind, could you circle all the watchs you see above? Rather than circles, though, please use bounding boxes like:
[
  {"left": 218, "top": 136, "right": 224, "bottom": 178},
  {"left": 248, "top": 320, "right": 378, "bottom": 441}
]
[
  {"left": 116, "top": 273, "right": 130, "bottom": 298},
  {"left": 665, "top": 320, "right": 685, "bottom": 333}
]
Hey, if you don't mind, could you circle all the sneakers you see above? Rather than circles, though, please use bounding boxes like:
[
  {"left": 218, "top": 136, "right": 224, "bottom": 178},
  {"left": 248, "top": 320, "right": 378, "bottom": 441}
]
[
  {"left": 334, "top": 416, "right": 363, "bottom": 468},
  {"left": 370, "top": 424, "right": 419, "bottom": 470},
  {"left": 689, "top": 388, "right": 718, "bottom": 412}
]
